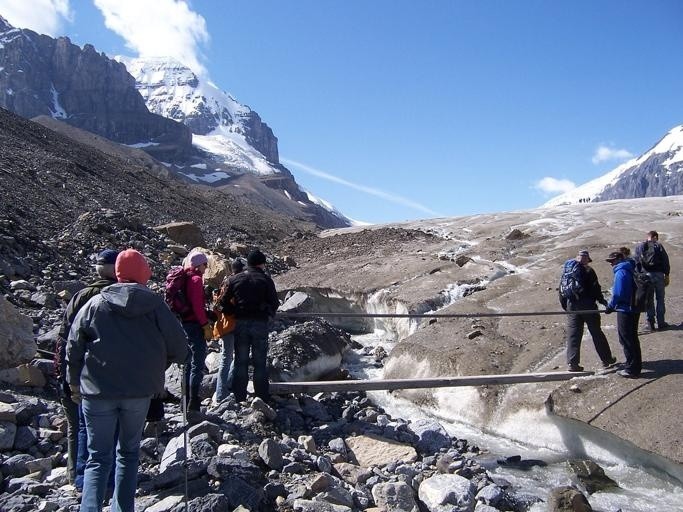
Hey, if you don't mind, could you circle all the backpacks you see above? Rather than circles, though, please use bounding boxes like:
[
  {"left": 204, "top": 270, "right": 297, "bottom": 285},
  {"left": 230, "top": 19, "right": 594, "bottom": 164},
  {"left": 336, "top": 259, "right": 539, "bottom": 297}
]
[
  {"left": 165, "top": 267, "right": 197, "bottom": 313},
  {"left": 641, "top": 241, "right": 659, "bottom": 266},
  {"left": 632, "top": 270, "right": 654, "bottom": 312},
  {"left": 559, "top": 261, "right": 589, "bottom": 299}
]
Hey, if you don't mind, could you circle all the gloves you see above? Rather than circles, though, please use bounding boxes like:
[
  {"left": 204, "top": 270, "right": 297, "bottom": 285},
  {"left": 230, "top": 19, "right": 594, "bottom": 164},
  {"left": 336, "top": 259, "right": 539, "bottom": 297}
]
[
  {"left": 203, "top": 323, "right": 213, "bottom": 342},
  {"left": 664, "top": 275, "right": 669, "bottom": 286},
  {"left": 605, "top": 307, "right": 612, "bottom": 314},
  {"left": 68, "top": 383, "right": 82, "bottom": 405},
  {"left": 599, "top": 299, "right": 608, "bottom": 306},
  {"left": 560, "top": 296, "right": 567, "bottom": 311}
]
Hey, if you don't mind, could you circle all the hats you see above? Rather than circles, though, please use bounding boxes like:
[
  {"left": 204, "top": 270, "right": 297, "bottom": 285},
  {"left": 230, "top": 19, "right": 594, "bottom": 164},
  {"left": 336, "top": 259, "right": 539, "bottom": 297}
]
[
  {"left": 115, "top": 250, "right": 152, "bottom": 284},
  {"left": 232, "top": 257, "right": 248, "bottom": 272},
  {"left": 578, "top": 250, "right": 592, "bottom": 262},
  {"left": 191, "top": 253, "right": 208, "bottom": 266},
  {"left": 98, "top": 249, "right": 118, "bottom": 264},
  {"left": 248, "top": 250, "right": 265, "bottom": 265},
  {"left": 606, "top": 252, "right": 623, "bottom": 259}
]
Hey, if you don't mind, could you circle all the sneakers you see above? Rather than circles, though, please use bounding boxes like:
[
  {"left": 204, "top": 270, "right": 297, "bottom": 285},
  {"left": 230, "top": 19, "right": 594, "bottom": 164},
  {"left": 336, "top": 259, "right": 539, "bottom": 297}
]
[
  {"left": 568, "top": 320, "right": 672, "bottom": 378},
  {"left": 188, "top": 400, "right": 200, "bottom": 411}
]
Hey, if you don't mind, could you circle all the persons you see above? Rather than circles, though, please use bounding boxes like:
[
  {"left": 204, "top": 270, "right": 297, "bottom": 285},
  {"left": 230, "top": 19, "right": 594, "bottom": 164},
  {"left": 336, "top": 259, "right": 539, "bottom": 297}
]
[
  {"left": 604, "top": 229, "right": 671, "bottom": 378},
  {"left": 212, "top": 257, "right": 244, "bottom": 405},
  {"left": 558, "top": 249, "right": 617, "bottom": 372},
  {"left": 220, "top": 249, "right": 281, "bottom": 408},
  {"left": 54, "top": 247, "right": 192, "bottom": 512},
  {"left": 173, "top": 250, "right": 214, "bottom": 414}
]
